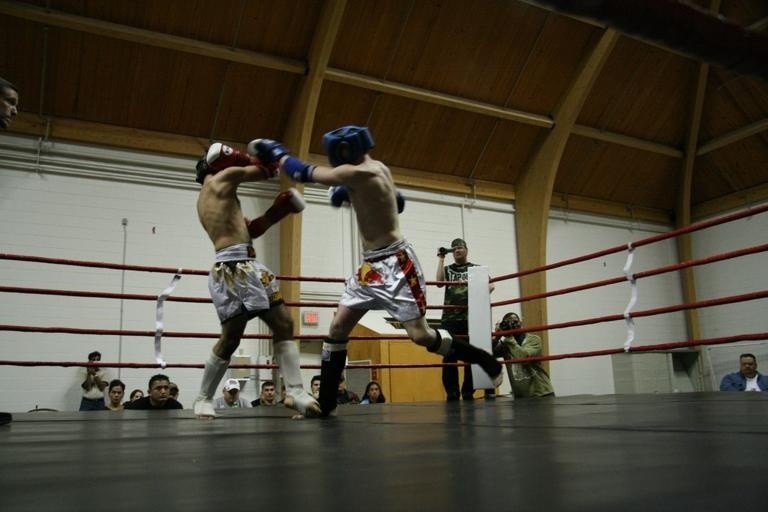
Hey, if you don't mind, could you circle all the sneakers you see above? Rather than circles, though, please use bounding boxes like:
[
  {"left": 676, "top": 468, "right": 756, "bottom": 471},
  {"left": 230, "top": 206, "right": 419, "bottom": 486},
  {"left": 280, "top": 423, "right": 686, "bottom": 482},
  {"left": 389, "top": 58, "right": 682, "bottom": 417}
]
[
  {"left": 447, "top": 391, "right": 460, "bottom": 402},
  {"left": 462, "top": 391, "right": 473, "bottom": 400}
]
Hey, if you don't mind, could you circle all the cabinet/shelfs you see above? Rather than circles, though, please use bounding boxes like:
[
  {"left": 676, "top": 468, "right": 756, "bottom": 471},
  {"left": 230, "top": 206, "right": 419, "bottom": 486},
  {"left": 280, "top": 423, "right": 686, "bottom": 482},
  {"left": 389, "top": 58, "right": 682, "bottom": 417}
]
[{"left": 608, "top": 346, "right": 706, "bottom": 395}]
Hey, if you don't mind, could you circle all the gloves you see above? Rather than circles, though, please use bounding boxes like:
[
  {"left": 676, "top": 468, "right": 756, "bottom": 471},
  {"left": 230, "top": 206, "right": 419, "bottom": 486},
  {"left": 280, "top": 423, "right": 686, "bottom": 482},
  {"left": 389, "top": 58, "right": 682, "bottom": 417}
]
[
  {"left": 328, "top": 184, "right": 350, "bottom": 207},
  {"left": 207, "top": 143, "right": 250, "bottom": 170},
  {"left": 247, "top": 137, "right": 288, "bottom": 166},
  {"left": 266, "top": 188, "right": 306, "bottom": 223}
]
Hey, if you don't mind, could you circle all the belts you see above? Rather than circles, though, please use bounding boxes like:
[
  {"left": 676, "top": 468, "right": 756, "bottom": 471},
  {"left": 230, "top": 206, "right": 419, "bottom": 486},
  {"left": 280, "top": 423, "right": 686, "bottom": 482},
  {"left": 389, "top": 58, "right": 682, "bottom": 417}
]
[{"left": 83, "top": 396, "right": 104, "bottom": 402}]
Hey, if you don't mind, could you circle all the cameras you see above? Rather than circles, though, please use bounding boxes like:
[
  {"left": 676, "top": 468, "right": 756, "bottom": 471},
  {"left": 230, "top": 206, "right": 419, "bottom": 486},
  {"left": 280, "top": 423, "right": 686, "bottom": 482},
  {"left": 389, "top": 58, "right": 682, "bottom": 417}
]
[
  {"left": 439, "top": 246, "right": 454, "bottom": 254},
  {"left": 499, "top": 319, "right": 520, "bottom": 332}
]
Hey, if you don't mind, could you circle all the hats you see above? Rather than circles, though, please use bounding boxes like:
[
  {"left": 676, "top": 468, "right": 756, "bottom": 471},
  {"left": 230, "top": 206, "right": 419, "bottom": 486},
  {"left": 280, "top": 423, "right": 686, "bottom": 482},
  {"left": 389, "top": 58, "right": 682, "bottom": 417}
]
[
  {"left": 451, "top": 238, "right": 466, "bottom": 248},
  {"left": 223, "top": 378, "right": 240, "bottom": 392}
]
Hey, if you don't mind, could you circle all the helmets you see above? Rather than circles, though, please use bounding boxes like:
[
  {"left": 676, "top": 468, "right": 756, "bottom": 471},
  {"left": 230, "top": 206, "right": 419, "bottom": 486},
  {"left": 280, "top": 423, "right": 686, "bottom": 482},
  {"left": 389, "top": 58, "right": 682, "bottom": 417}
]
[
  {"left": 195, "top": 157, "right": 214, "bottom": 185},
  {"left": 323, "top": 125, "right": 374, "bottom": 167}
]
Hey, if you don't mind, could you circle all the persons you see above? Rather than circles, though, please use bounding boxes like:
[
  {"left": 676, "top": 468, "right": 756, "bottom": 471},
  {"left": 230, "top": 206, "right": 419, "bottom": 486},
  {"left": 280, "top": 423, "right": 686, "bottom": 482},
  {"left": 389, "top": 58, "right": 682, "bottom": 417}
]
[
  {"left": 214, "top": 378, "right": 254, "bottom": 408},
  {"left": 490, "top": 311, "right": 555, "bottom": 398},
  {"left": 246, "top": 124, "right": 502, "bottom": 420},
  {"left": 434, "top": 238, "right": 496, "bottom": 405},
  {"left": 278, "top": 385, "right": 287, "bottom": 406},
  {"left": 357, "top": 382, "right": 385, "bottom": 403},
  {"left": 193, "top": 142, "right": 324, "bottom": 418},
  {"left": 168, "top": 382, "right": 180, "bottom": 401},
  {"left": 255, "top": 382, "right": 287, "bottom": 409},
  {"left": 103, "top": 379, "right": 127, "bottom": 411},
  {"left": 0, "top": 76, "right": 20, "bottom": 130},
  {"left": 79, "top": 350, "right": 112, "bottom": 410},
  {"left": 128, "top": 389, "right": 144, "bottom": 402},
  {"left": 129, "top": 374, "right": 184, "bottom": 408},
  {"left": 308, "top": 375, "right": 321, "bottom": 399},
  {"left": 336, "top": 373, "right": 362, "bottom": 403},
  {"left": 718, "top": 352, "right": 767, "bottom": 392}
]
